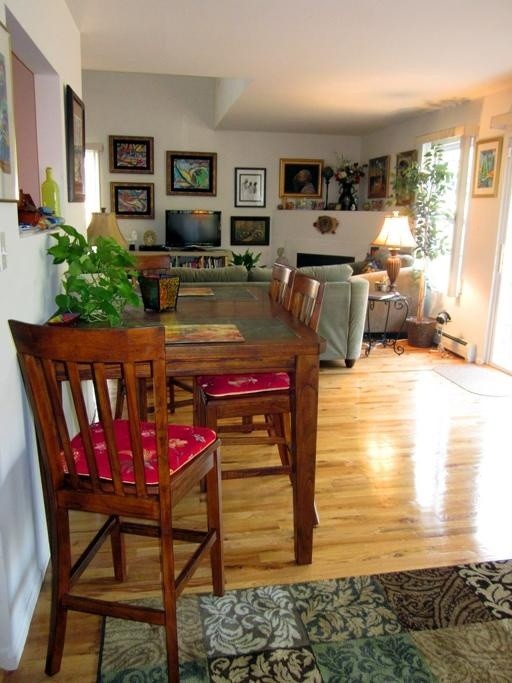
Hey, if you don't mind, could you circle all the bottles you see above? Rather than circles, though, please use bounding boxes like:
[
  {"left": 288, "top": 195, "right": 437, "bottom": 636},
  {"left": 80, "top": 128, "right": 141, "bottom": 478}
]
[{"left": 41, "top": 168, "right": 60, "bottom": 218}]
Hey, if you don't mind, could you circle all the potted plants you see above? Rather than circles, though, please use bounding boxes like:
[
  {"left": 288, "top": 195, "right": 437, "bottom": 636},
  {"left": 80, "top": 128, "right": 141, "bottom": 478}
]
[
  {"left": 46, "top": 223, "right": 143, "bottom": 329},
  {"left": 392, "top": 142, "right": 454, "bottom": 347}
]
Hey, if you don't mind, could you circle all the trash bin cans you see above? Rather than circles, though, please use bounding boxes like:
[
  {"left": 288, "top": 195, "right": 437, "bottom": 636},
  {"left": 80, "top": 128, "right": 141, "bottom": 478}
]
[{"left": 405, "top": 315, "right": 437, "bottom": 348}]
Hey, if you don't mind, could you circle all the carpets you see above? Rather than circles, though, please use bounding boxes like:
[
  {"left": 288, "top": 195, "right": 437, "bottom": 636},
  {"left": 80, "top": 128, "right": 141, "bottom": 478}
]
[{"left": 98, "top": 559, "right": 511, "bottom": 679}]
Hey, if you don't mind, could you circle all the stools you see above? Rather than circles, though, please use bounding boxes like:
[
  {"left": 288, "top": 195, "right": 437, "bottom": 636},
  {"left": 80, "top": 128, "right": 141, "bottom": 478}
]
[{"left": 365, "top": 295, "right": 408, "bottom": 356}]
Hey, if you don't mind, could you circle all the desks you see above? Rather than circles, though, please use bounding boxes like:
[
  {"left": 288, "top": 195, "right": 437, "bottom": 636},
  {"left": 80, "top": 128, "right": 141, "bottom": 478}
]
[{"left": 47, "top": 286, "right": 326, "bottom": 564}]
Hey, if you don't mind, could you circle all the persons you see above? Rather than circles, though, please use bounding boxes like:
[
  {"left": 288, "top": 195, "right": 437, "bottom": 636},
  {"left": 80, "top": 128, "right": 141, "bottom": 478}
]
[
  {"left": 240, "top": 179, "right": 259, "bottom": 200},
  {"left": 292, "top": 169, "right": 315, "bottom": 194}
]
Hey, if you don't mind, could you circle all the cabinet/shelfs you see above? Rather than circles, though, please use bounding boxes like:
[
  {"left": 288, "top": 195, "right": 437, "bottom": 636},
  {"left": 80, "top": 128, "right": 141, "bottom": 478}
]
[{"left": 128, "top": 249, "right": 233, "bottom": 269}]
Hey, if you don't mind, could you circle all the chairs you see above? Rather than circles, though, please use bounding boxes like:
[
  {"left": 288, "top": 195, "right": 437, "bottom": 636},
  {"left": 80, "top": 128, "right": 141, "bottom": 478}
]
[
  {"left": 242, "top": 263, "right": 296, "bottom": 432},
  {"left": 7, "top": 319, "right": 225, "bottom": 683},
  {"left": 191, "top": 273, "right": 321, "bottom": 529}
]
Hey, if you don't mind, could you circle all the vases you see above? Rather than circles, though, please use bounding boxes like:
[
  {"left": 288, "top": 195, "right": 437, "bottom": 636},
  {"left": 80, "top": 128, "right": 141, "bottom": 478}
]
[{"left": 338, "top": 185, "right": 358, "bottom": 209}]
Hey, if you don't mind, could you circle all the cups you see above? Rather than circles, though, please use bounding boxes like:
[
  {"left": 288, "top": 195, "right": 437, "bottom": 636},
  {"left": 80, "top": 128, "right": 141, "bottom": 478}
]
[{"left": 374, "top": 282, "right": 389, "bottom": 293}]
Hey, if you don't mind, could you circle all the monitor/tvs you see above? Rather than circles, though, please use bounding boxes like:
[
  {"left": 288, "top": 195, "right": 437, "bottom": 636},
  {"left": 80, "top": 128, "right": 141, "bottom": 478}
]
[{"left": 165, "top": 210, "right": 221, "bottom": 252}]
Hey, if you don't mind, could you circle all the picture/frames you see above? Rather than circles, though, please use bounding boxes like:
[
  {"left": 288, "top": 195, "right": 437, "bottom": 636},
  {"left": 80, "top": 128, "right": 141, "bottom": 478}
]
[
  {"left": 230, "top": 215, "right": 271, "bottom": 245},
  {"left": 280, "top": 158, "right": 324, "bottom": 198},
  {"left": 396, "top": 149, "right": 418, "bottom": 206},
  {"left": 235, "top": 167, "right": 266, "bottom": 208},
  {"left": 0, "top": 21, "right": 19, "bottom": 202},
  {"left": 472, "top": 136, "right": 504, "bottom": 199},
  {"left": 66, "top": 84, "right": 85, "bottom": 202},
  {"left": 110, "top": 182, "right": 155, "bottom": 220},
  {"left": 109, "top": 136, "right": 154, "bottom": 175},
  {"left": 166, "top": 151, "right": 217, "bottom": 197},
  {"left": 368, "top": 155, "right": 390, "bottom": 199}
]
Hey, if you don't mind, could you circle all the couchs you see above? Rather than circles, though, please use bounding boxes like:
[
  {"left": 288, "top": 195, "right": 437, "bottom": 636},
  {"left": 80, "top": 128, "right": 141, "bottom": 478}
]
[
  {"left": 148, "top": 265, "right": 369, "bottom": 367},
  {"left": 347, "top": 250, "right": 430, "bottom": 332}
]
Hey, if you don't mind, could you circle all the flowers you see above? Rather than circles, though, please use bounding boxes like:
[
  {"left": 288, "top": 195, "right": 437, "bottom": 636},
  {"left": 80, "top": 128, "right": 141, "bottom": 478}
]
[{"left": 334, "top": 152, "right": 368, "bottom": 185}]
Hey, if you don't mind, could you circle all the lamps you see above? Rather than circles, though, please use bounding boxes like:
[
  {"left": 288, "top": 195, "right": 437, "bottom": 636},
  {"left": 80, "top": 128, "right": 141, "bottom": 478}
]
[
  {"left": 371, "top": 211, "right": 419, "bottom": 294},
  {"left": 87, "top": 208, "right": 126, "bottom": 247}
]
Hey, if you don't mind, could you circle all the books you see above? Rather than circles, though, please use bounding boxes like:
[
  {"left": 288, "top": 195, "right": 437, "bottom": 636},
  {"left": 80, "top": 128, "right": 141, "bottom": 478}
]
[{"left": 180, "top": 257, "right": 224, "bottom": 269}]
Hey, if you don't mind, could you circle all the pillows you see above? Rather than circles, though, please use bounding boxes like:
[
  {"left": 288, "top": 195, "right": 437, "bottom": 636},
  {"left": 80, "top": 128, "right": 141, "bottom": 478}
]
[{"left": 351, "top": 253, "right": 383, "bottom": 273}]
